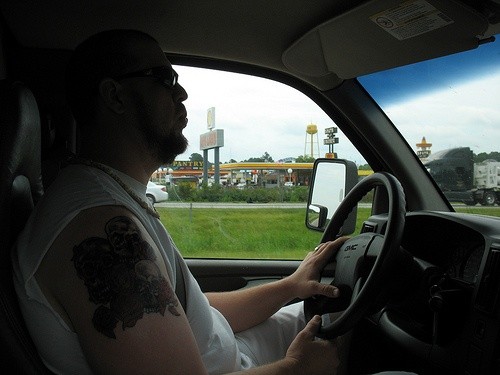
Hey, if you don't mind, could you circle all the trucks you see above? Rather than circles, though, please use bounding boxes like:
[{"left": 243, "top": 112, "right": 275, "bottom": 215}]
[{"left": 423, "top": 146, "right": 500, "bottom": 207}]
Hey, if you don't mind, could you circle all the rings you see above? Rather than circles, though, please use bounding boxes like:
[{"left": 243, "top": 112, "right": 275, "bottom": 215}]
[{"left": 314, "top": 246, "right": 319, "bottom": 251}]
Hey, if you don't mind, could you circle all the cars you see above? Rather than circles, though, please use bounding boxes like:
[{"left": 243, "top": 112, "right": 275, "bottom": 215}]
[
  {"left": 145, "top": 180, "right": 169, "bottom": 206},
  {"left": 284, "top": 181, "right": 293, "bottom": 187}
]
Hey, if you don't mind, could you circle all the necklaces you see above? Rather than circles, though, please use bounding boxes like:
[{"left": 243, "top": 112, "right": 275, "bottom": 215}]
[{"left": 70, "top": 156, "right": 162, "bottom": 223}]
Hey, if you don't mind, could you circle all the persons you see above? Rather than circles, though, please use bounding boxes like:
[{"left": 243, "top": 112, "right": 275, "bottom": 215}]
[
  {"left": 164, "top": 171, "right": 309, "bottom": 190},
  {"left": 8, "top": 26, "right": 354, "bottom": 374}
]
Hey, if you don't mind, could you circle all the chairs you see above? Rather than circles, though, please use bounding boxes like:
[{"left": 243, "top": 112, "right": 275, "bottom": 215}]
[{"left": 0, "top": 86, "right": 88, "bottom": 375}]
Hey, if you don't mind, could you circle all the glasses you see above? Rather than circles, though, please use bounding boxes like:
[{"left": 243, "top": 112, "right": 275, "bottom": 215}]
[{"left": 103, "top": 67, "right": 179, "bottom": 89}]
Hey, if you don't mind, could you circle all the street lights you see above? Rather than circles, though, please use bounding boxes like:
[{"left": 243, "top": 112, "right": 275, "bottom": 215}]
[{"left": 287, "top": 167, "right": 292, "bottom": 182}]
